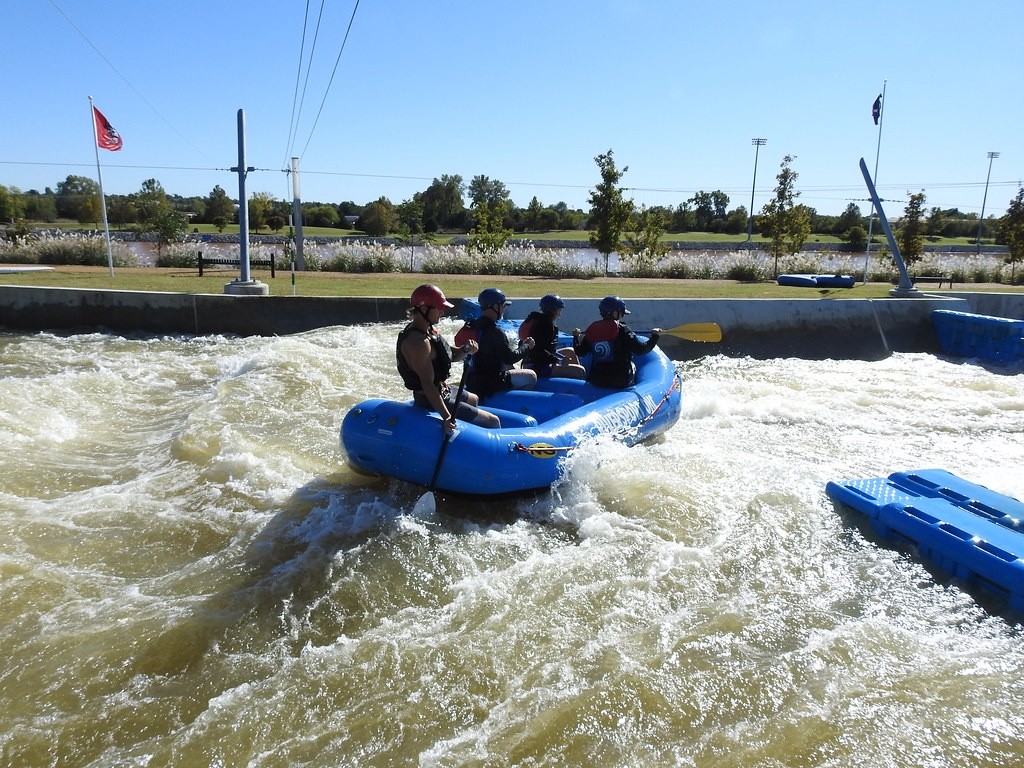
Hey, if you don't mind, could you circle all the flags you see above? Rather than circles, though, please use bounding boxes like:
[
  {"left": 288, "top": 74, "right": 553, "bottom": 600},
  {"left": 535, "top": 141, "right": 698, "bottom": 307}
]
[
  {"left": 871, "top": 94, "right": 883, "bottom": 126},
  {"left": 91, "top": 105, "right": 124, "bottom": 152}
]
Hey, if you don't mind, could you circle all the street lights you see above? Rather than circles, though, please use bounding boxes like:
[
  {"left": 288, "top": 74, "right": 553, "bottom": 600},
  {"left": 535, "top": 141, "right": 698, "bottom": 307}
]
[
  {"left": 974, "top": 151, "right": 1000, "bottom": 245},
  {"left": 747, "top": 138, "right": 767, "bottom": 241}
]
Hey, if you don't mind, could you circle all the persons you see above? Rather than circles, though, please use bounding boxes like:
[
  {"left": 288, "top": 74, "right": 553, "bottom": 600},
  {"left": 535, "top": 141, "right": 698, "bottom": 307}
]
[
  {"left": 573, "top": 295, "right": 663, "bottom": 389},
  {"left": 457, "top": 288, "right": 538, "bottom": 393},
  {"left": 519, "top": 295, "right": 586, "bottom": 379},
  {"left": 395, "top": 284, "right": 501, "bottom": 435}
]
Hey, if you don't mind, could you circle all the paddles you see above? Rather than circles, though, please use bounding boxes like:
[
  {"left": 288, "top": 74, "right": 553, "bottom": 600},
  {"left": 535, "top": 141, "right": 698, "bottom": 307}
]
[
  {"left": 579, "top": 322, "right": 723, "bottom": 342},
  {"left": 411, "top": 352, "right": 473, "bottom": 517}
]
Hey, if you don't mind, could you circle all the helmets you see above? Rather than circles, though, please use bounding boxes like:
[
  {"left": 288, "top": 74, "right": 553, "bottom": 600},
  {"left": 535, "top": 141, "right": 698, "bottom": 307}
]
[
  {"left": 539, "top": 294, "right": 565, "bottom": 309},
  {"left": 411, "top": 284, "right": 455, "bottom": 310},
  {"left": 478, "top": 289, "right": 512, "bottom": 307},
  {"left": 599, "top": 296, "right": 632, "bottom": 314}
]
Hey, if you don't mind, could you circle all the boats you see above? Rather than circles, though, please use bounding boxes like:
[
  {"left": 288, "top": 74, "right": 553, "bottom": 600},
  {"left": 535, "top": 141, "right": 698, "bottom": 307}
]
[
  {"left": 340, "top": 334, "right": 683, "bottom": 495},
  {"left": 778, "top": 274, "right": 856, "bottom": 287}
]
